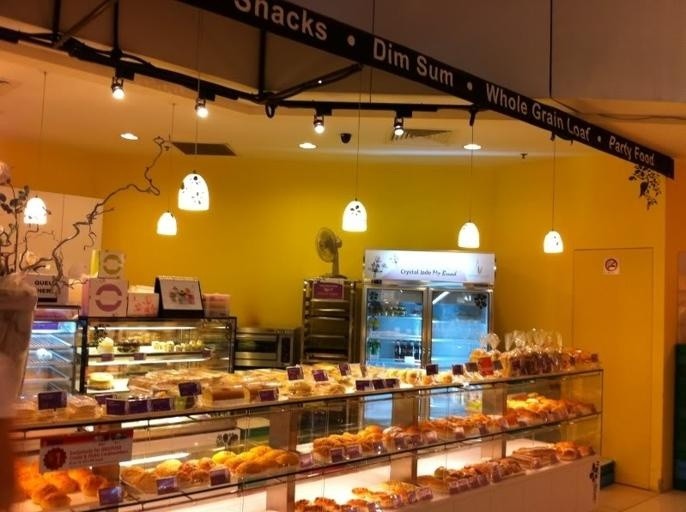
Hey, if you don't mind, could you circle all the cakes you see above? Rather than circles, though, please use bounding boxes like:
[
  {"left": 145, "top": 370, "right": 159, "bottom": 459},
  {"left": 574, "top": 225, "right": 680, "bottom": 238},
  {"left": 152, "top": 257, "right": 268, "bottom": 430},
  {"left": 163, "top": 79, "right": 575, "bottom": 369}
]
[
  {"left": 96, "top": 337, "right": 144, "bottom": 362},
  {"left": 150, "top": 338, "right": 204, "bottom": 353}
]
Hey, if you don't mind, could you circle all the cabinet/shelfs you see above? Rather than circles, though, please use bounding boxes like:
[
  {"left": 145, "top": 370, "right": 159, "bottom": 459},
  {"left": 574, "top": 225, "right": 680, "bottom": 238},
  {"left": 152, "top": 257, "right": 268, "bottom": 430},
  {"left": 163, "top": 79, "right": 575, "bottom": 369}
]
[
  {"left": 0, "top": 370, "right": 604, "bottom": 512},
  {"left": 24, "top": 305, "right": 76, "bottom": 398},
  {"left": 300, "top": 277, "right": 357, "bottom": 365},
  {"left": 76, "top": 319, "right": 234, "bottom": 401}
]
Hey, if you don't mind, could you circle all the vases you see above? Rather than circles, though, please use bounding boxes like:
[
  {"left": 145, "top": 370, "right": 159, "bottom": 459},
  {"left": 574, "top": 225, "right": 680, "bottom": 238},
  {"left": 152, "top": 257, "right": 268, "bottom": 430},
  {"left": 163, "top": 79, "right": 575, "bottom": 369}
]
[{"left": 0, "top": 268, "right": 40, "bottom": 403}]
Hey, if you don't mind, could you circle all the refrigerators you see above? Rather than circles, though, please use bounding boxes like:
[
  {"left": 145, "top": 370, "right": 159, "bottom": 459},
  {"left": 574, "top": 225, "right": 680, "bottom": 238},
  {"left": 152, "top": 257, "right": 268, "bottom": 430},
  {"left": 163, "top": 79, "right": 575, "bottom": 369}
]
[{"left": 357, "top": 282, "right": 495, "bottom": 426}]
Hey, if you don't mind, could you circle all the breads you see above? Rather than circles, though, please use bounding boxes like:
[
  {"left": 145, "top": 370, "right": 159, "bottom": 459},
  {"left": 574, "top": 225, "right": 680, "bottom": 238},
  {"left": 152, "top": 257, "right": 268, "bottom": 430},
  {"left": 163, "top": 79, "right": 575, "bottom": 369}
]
[
  {"left": 513, "top": 438, "right": 592, "bottom": 471},
  {"left": 96, "top": 369, "right": 277, "bottom": 417},
  {"left": 420, "top": 413, "right": 492, "bottom": 435},
  {"left": 246, "top": 356, "right": 454, "bottom": 400},
  {"left": 295, "top": 480, "right": 432, "bottom": 511},
  {"left": 417, "top": 456, "right": 527, "bottom": 497},
  {"left": 507, "top": 392, "right": 595, "bottom": 424},
  {"left": 469, "top": 348, "right": 599, "bottom": 382},
  {"left": 312, "top": 425, "right": 422, "bottom": 465},
  {"left": 124, "top": 445, "right": 298, "bottom": 494},
  {"left": 10, "top": 394, "right": 114, "bottom": 426},
  {"left": 13, "top": 458, "right": 111, "bottom": 512}
]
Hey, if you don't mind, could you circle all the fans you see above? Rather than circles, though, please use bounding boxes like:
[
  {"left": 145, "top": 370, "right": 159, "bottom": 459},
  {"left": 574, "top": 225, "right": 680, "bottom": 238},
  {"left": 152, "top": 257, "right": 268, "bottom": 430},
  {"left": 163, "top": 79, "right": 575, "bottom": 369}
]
[{"left": 313, "top": 227, "right": 346, "bottom": 279}]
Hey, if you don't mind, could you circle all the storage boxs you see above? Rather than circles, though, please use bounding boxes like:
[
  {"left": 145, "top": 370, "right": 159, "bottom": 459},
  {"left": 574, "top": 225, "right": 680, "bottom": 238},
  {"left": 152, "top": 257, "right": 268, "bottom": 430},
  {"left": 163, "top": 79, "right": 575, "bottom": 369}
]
[
  {"left": 124, "top": 290, "right": 160, "bottom": 317},
  {"left": 77, "top": 276, "right": 129, "bottom": 318},
  {"left": 88, "top": 248, "right": 127, "bottom": 280},
  {"left": 200, "top": 292, "right": 231, "bottom": 318}
]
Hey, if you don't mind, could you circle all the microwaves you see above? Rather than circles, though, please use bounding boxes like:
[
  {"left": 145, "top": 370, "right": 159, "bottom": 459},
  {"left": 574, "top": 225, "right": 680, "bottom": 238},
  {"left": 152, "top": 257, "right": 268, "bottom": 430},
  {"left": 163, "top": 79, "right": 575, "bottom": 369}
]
[{"left": 234, "top": 327, "right": 301, "bottom": 368}]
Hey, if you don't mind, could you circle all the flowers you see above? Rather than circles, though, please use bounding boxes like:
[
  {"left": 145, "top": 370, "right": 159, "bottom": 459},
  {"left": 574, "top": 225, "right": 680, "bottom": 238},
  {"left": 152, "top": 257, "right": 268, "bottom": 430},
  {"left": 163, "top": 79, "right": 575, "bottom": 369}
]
[{"left": 0, "top": 159, "right": 31, "bottom": 274}]
